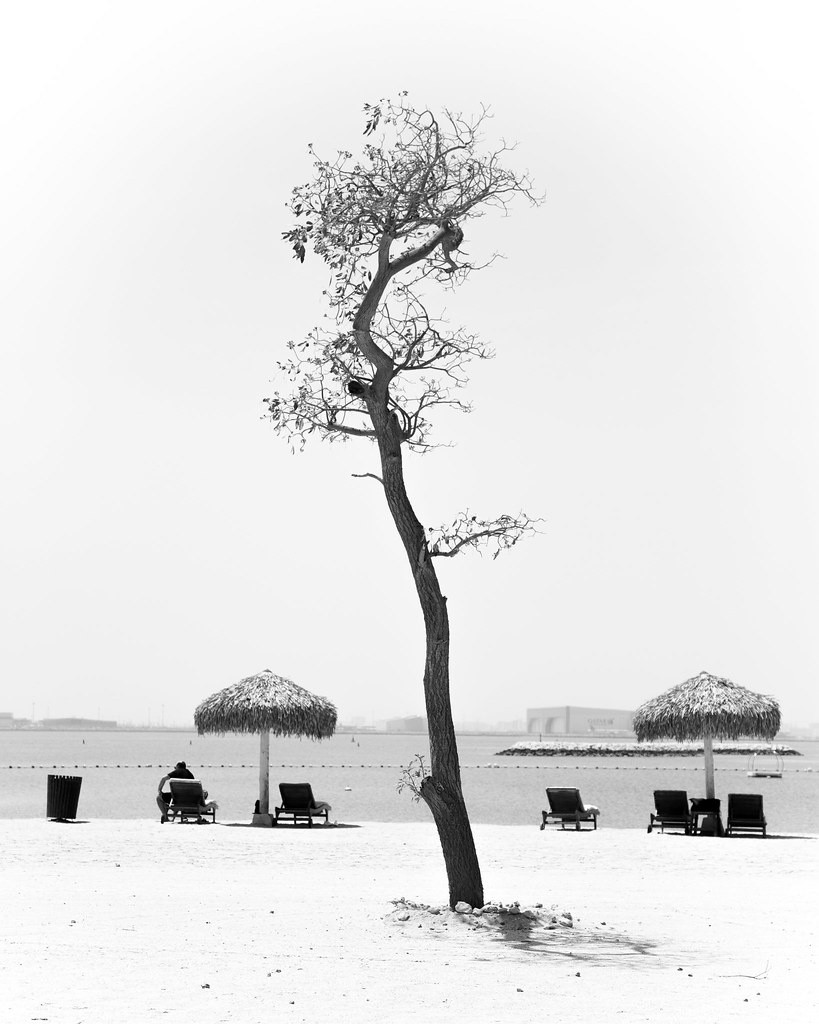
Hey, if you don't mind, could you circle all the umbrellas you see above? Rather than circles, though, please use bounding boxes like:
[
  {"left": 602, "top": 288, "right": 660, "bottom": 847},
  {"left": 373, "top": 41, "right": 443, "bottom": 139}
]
[
  {"left": 195, "top": 668, "right": 336, "bottom": 813},
  {"left": 633, "top": 672, "right": 781, "bottom": 818}
]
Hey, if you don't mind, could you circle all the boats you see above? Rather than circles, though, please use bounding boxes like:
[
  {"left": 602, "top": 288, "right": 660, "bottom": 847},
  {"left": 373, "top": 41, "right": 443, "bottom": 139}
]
[{"left": 748, "top": 746, "right": 784, "bottom": 778}]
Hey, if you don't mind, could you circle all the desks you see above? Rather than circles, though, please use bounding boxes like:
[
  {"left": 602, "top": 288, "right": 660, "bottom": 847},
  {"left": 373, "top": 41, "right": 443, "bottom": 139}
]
[{"left": 690, "top": 812, "right": 719, "bottom": 836}]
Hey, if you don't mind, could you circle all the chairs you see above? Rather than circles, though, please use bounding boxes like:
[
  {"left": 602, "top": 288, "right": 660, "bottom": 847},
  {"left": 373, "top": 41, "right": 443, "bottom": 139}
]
[
  {"left": 276, "top": 783, "right": 331, "bottom": 828},
  {"left": 725, "top": 794, "right": 767, "bottom": 836},
  {"left": 540, "top": 788, "right": 599, "bottom": 831},
  {"left": 647, "top": 790, "right": 696, "bottom": 835},
  {"left": 164, "top": 778, "right": 218, "bottom": 823}
]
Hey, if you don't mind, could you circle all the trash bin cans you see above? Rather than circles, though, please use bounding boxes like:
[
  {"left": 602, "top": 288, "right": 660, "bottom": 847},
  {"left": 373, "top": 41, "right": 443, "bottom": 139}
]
[{"left": 46, "top": 774, "right": 83, "bottom": 823}]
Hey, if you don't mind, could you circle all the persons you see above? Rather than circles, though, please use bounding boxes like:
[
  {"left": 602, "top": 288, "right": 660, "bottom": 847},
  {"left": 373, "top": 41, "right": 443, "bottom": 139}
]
[{"left": 156, "top": 759, "right": 199, "bottom": 824}]
